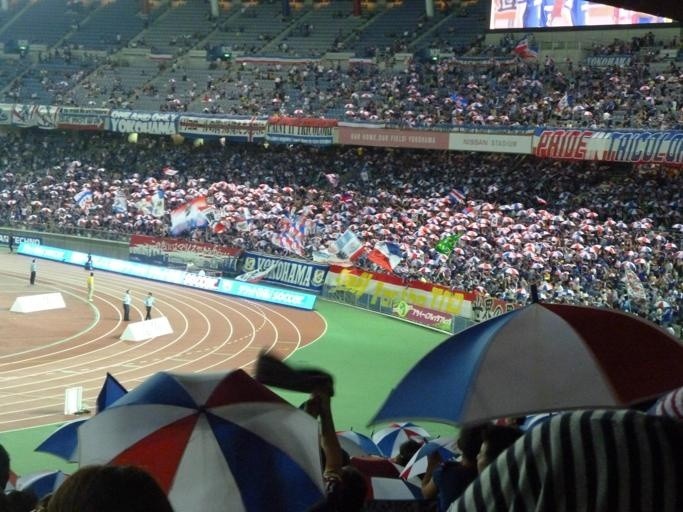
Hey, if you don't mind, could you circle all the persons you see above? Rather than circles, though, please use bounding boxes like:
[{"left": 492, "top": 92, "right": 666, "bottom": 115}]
[{"left": 1, "top": 1, "right": 682, "bottom": 512}]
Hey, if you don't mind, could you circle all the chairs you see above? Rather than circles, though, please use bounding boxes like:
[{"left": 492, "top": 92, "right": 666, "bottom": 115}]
[{"left": 0, "top": 1, "right": 683, "bottom": 132}]
